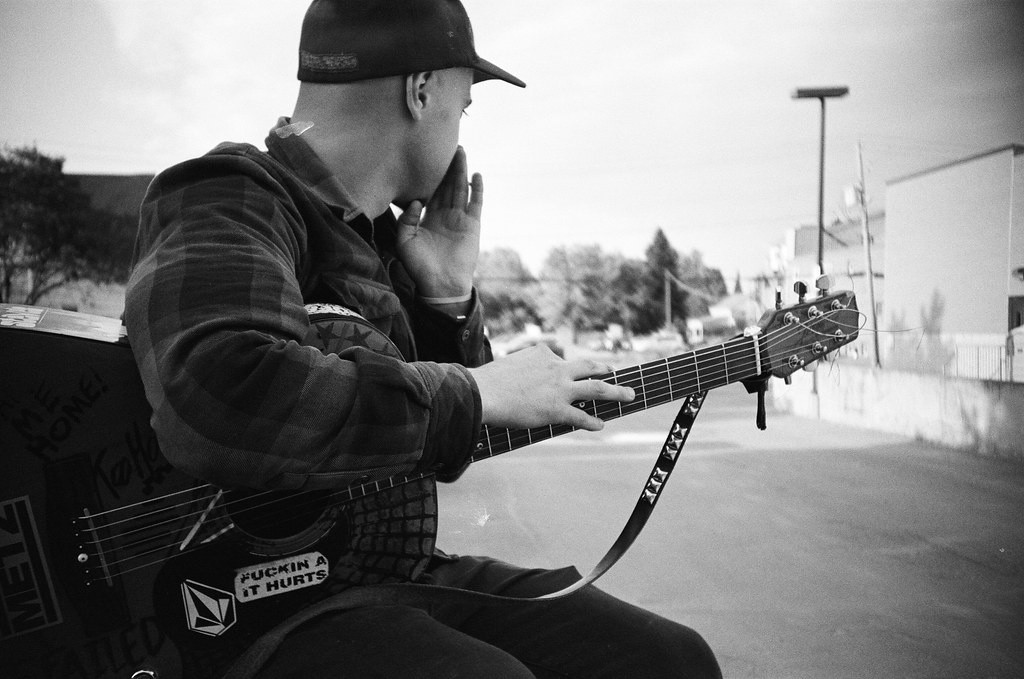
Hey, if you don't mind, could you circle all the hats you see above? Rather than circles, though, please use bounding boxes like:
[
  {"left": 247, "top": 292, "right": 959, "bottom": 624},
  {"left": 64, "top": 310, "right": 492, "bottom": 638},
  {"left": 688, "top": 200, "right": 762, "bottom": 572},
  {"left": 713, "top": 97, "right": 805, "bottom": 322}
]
[{"left": 297, "top": 0, "right": 526, "bottom": 90}]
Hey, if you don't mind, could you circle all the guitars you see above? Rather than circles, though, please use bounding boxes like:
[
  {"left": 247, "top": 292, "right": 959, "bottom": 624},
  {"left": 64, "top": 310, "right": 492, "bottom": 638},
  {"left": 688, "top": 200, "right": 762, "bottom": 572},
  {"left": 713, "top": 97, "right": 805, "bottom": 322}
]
[{"left": 1, "top": 275, "right": 862, "bottom": 679}]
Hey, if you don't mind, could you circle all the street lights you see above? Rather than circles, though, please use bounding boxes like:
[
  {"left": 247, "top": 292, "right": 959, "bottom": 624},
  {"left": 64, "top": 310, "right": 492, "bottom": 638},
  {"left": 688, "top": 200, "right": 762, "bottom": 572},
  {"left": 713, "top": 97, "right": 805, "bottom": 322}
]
[{"left": 792, "top": 85, "right": 849, "bottom": 277}]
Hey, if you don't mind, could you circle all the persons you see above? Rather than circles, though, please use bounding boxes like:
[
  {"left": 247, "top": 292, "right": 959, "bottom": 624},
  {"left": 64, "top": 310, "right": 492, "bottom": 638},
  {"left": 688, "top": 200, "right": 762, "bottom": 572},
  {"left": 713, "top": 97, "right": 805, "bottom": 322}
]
[{"left": 120, "top": 0, "right": 724, "bottom": 679}]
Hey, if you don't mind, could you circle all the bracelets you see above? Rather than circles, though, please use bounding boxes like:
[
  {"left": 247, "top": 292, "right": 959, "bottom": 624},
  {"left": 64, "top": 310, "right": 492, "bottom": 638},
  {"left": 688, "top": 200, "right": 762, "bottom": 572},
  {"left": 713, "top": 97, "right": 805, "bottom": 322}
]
[{"left": 422, "top": 292, "right": 472, "bottom": 306}]
